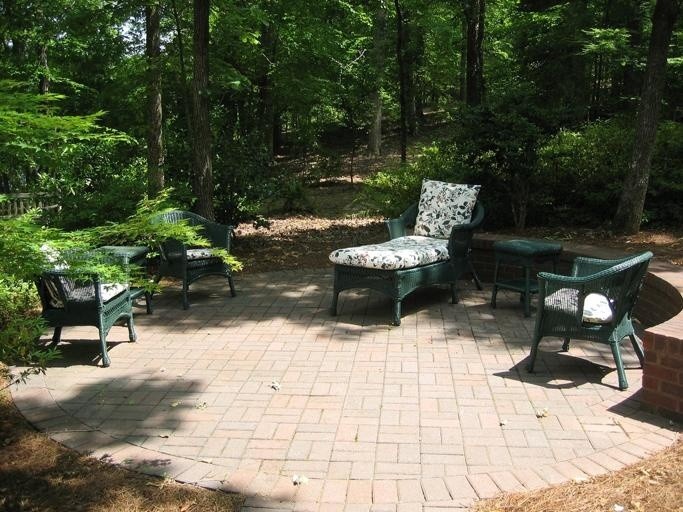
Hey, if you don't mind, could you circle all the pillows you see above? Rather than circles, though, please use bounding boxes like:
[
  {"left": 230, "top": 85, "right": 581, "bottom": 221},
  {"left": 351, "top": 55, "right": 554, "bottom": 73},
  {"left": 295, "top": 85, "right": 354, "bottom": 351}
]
[
  {"left": 414, "top": 177, "right": 482, "bottom": 240},
  {"left": 36, "top": 242, "right": 75, "bottom": 301}
]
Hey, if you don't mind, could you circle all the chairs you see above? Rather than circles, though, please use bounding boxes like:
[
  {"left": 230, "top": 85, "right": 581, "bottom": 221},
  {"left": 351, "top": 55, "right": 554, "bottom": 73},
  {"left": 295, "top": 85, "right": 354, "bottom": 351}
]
[
  {"left": 527, "top": 251, "right": 654, "bottom": 390},
  {"left": 34, "top": 269, "right": 137, "bottom": 367},
  {"left": 151, "top": 210, "right": 236, "bottom": 311}
]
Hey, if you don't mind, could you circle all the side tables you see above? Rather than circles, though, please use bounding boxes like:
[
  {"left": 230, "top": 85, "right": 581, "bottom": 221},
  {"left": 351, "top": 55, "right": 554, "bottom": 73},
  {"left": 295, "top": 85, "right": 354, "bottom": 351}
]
[
  {"left": 491, "top": 240, "right": 564, "bottom": 318},
  {"left": 91, "top": 244, "right": 152, "bottom": 317}
]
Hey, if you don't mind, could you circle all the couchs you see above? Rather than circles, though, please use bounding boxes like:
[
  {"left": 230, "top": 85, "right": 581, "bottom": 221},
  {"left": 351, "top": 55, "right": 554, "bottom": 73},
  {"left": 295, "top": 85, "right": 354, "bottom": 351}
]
[{"left": 328, "top": 183, "right": 488, "bottom": 325}]
